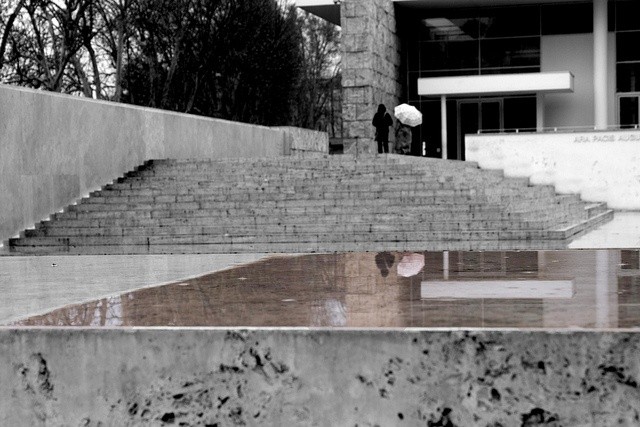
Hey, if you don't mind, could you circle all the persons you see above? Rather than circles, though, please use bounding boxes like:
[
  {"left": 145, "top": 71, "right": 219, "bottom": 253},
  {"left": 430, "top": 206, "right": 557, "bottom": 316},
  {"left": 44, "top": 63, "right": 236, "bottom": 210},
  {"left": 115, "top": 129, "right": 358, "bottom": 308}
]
[
  {"left": 394, "top": 117, "right": 414, "bottom": 155},
  {"left": 371, "top": 104, "right": 393, "bottom": 154}
]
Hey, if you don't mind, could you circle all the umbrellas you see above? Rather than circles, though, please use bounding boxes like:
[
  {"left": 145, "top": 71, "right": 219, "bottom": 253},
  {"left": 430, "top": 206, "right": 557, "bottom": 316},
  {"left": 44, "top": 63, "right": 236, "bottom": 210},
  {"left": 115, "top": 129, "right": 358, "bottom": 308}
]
[{"left": 393, "top": 102, "right": 422, "bottom": 127}]
